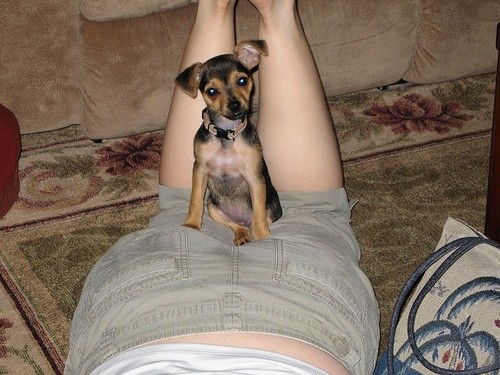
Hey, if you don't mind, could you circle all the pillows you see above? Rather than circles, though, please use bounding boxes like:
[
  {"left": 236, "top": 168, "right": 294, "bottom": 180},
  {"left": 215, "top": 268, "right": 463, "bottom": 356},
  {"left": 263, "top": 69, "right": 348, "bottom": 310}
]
[
  {"left": 370, "top": 215, "right": 500, "bottom": 374},
  {"left": 78, "top": 1, "right": 500, "bottom": 144}
]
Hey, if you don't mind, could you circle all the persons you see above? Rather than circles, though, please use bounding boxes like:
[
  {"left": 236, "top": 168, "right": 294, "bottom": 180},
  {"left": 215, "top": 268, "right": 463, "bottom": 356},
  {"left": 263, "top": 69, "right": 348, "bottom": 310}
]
[{"left": 59, "top": 0, "right": 382, "bottom": 375}]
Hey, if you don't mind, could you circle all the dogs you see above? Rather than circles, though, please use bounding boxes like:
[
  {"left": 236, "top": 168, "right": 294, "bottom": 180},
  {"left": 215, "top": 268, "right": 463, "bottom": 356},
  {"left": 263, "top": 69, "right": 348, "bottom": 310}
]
[{"left": 173, "top": 38, "right": 283, "bottom": 245}]
